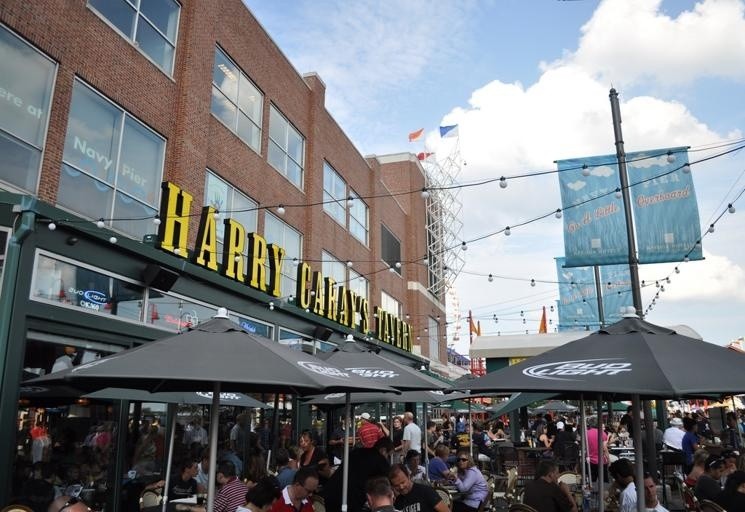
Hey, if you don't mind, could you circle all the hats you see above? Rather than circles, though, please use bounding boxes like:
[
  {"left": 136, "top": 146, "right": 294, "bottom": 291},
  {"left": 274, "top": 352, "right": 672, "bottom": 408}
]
[
  {"left": 362, "top": 413, "right": 370, "bottom": 420},
  {"left": 556, "top": 422, "right": 564, "bottom": 430},
  {"left": 670, "top": 418, "right": 683, "bottom": 426}
]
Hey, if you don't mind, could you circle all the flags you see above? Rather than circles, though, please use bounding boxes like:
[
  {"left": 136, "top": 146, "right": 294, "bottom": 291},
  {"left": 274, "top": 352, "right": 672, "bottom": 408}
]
[
  {"left": 409, "top": 128, "right": 424, "bottom": 142},
  {"left": 418, "top": 152, "right": 435, "bottom": 163},
  {"left": 440, "top": 124, "right": 458, "bottom": 138}
]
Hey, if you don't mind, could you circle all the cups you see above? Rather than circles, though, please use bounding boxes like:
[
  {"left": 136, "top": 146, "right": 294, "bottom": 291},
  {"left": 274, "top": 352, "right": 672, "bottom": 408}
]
[{"left": 197, "top": 492, "right": 204, "bottom": 505}]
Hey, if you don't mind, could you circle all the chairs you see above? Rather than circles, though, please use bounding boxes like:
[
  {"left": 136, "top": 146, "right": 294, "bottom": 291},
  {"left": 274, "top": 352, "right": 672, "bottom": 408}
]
[
  {"left": 656, "top": 446, "right": 724, "bottom": 511},
  {"left": 139, "top": 489, "right": 161, "bottom": 510},
  {"left": 427, "top": 445, "right": 638, "bottom": 510},
  {"left": 310, "top": 495, "right": 327, "bottom": 511}
]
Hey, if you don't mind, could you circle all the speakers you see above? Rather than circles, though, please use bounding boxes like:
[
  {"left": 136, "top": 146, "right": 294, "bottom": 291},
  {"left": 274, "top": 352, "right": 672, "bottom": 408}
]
[
  {"left": 316, "top": 326, "right": 334, "bottom": 341},
  {"left": 142, "top": 263, "right": 180, "bottom": 292}
]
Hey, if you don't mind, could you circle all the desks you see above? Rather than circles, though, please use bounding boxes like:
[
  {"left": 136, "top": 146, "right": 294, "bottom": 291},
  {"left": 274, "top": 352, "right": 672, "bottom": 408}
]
[{"left": 171, "top": 493, "right": 207, "bottom": 511}]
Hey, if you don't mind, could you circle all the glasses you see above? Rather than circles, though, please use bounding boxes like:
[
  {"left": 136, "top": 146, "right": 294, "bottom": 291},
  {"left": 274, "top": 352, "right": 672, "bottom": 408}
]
[{"left": 457, "top": 457, "right": 469, "bottom": 462}]
